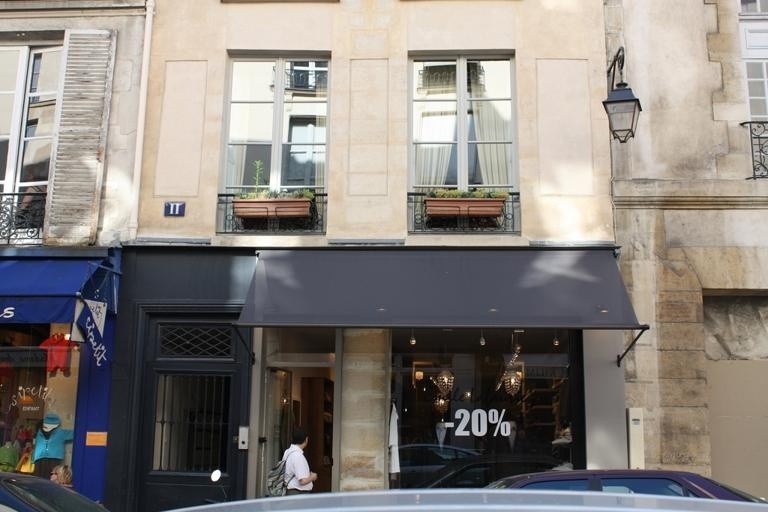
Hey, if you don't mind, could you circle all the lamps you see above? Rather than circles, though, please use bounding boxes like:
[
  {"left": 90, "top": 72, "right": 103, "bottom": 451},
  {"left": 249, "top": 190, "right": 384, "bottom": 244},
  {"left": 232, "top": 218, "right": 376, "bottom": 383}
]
[
  {"left": 409, "top": 328, "right": 417, "bottom": 345},
  {"left": 602, "top": 47, "right": 642, "bottom": 144},
  {"left": 504, "top": 353, "right": 523, "bottom": 398},
  {"left": 553, "top": 329, "right": 560, "bottom": 346},
  {"left": 415, "top": 371, "right": 424, "bottom": 380},
  {"left": 435, "top": 397, "right": 450, "bottom": 415},
  {"left": 435, "top": 370, "right": 454, "bottom": 396},
  {"left": 480, "top": 328, "right": 486, "bottom": 346}
]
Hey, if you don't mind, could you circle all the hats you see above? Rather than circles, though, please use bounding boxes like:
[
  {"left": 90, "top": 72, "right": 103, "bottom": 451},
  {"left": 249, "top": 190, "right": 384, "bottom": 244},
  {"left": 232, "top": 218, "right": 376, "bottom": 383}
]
[{"left": 43, "top": 413, "right": 60, "bottom": 425}]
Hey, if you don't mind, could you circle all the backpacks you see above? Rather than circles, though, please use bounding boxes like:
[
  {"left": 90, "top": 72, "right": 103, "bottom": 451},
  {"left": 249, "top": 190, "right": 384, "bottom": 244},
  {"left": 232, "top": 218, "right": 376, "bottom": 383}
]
[{"left": 267, "top": 451, "right": 302, "bottom": 497}]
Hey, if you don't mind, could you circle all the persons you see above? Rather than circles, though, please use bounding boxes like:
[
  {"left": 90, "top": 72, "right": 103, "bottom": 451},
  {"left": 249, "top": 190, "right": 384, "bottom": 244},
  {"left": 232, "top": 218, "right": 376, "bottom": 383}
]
[{"left": 283, "top": 427, "right": 318, "bottom": 495}]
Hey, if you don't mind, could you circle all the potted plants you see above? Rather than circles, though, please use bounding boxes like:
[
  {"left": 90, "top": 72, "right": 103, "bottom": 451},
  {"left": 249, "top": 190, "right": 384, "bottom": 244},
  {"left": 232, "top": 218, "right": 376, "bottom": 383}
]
[
  {"left": 423, "top": 189, "right": 508, "bottom": 216},
  {"left": 232, "top": 160, "right": 314, "bottom": 219}
]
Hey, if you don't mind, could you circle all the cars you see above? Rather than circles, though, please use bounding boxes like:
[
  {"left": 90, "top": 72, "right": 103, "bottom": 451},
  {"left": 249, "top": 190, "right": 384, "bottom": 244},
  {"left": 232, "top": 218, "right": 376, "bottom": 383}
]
[{"left": 337, "top": 444, "right": 576, "bottom": 489}]
